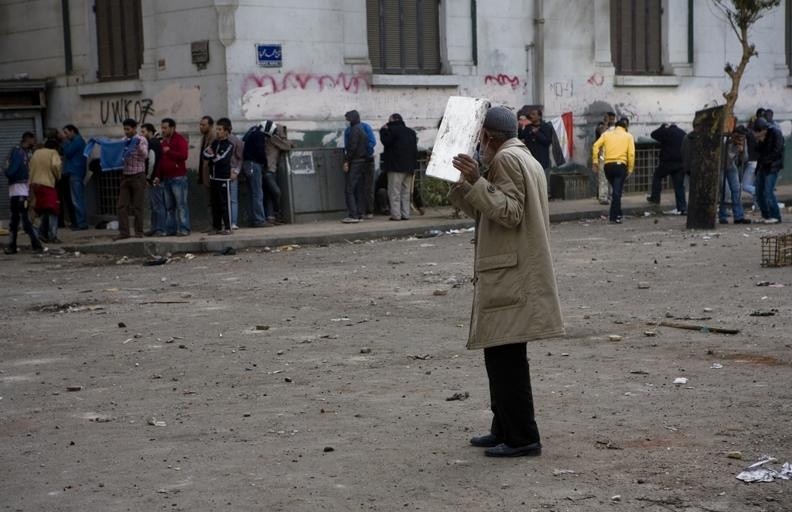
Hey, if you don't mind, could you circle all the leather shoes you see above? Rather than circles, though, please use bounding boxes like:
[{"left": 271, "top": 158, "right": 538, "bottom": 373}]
[
  {"left": 485, "top": 442, "right": 542, "bottom": 457},
  {"left": 469, "top": 435, "right": 499, "bottom": 445}
]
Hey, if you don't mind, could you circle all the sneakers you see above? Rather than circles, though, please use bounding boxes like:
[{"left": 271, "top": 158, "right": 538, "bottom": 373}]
[{"left": 342, "top": 217, "right": 359, "bottom": 223}]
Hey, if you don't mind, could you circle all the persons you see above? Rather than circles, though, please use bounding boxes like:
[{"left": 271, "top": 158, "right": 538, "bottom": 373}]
[
  {"left": 373, "top": 171, "right": 425, "bottom": 217},
  {"left": 595, "top": 112, "right": 616, "bottom": 206},
  {"left": 342, "top": 123, "right": 376, "bottom": 219},
  {"left": 1, "top": 111, "right": 190, "bottom": 257},
  {"left": 715, "top": 107, "right": 785, "bottom": 225},
  {"left": 591, "top": 120, "right": 635, "bottom": 224},
  {"left": 341, "top": 110, "right": 369, "bottom": 224},
  {"left": 380, "top": 113, "right": 418, "bottom": 221},
  {"left": 645, "top": 123, "right": 695, "bottom": 215},
  {"left": 517, "top": 112, "right": 530, "bottom": 131},
  {"left": 620, "top": 116, "right": 633, "bottom": 139},
  {"left": 425, "top": 103, "right": 568, "bottom": 457},
  {"left": 198, "top": 111, "right": 296, "bottom": 234},
  {"left": 519, "top": 104, "right": 556, "bottom": 202}
]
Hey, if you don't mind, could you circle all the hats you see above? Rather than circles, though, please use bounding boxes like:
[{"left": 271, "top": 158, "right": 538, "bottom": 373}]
[
  {"left": 753, "top": 117, "right": 769, "bottom": 126},
  {"left": 732, "top": 125, "right": 748, "bottom": 134},
  {"left": 484, "top": 108, "right": 517, "bottom": 131}
]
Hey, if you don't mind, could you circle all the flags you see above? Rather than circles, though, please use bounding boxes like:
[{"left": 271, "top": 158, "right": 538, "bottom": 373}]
[{"left": 544, "top": 112, "right": 573, "bottom": 169}]
